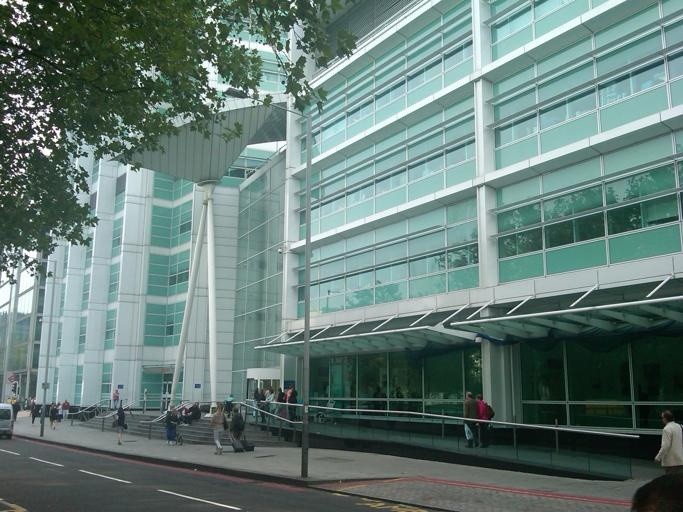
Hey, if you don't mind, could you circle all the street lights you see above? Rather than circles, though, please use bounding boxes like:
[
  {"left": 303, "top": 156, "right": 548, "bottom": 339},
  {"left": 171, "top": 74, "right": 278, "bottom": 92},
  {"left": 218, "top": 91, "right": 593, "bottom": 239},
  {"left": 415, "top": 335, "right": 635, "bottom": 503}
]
[{"left": 224, "top": 87, "right": 312, "bottom": 478}]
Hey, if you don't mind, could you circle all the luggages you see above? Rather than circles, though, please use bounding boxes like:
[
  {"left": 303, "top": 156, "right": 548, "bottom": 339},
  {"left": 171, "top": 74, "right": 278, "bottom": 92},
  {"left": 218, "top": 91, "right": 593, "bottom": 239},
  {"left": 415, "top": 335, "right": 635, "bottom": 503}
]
[
  {"left": 232, "top": 440, "right": 243, "bottom": 453},
  {"left": 243, "top": 440, "right": 255, "bottom": 452}
]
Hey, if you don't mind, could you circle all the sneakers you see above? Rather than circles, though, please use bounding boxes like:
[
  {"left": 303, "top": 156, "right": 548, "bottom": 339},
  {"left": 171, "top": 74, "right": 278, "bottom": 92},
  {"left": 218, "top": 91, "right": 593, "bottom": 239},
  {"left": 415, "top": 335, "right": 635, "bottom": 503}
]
[{"left": 218, "top": 445, "right": 223, "bottom": 454}]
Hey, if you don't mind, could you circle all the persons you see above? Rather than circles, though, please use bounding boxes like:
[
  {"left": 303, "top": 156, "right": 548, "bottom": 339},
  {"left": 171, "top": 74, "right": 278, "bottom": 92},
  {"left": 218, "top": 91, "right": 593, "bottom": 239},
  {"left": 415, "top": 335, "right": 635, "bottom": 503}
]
[
  {"left": 393, "top": 383, "right": 409, "bottom": 408},
  {"left": 251, "top": 386, "right": 298, "bottom": 422},
  {"left": 207, "top": 404, "right": 226, "bottom": 454},
  {"left": 186, "top": 403, "right": 200, "bottom": 423},
  {"left": 476, "top": 394, "right": 494, "bottom": 447},
  {"left": 229, "top": 406, "right": 244, "bottom": 440},
  {"left": 372, "top": 386, "right": 387, "bottom": 416},
  {"left": 5, "top": 395, "right": 69, "bottom": 430},
  {"left": 112, "top": 389, "right": 119, "bottom": 408},
  {"left": 462, "top": 392, "right": 479, "bottom": 447},
  {"left": 117, "top": 405, "right": 124, "bottom": 445},
  {"left": 177, "top": 405, "right": 188, "bottom": 426},
  {"left": 223, "top": 400, "right": 231, "bottom": 417},
  {"left": 631, "top": 472, "right": 682, "bottom": 511},
  {"left": 653, "top": 409, "right": 683, "bottom": 474}
]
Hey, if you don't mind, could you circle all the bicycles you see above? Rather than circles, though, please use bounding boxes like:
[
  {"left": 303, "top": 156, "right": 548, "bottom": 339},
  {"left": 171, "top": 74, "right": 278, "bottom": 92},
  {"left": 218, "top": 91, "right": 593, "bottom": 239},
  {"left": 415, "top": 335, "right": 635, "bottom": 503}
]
[{"left": 166, "top": 432, "right": 185, "bottom": 446}]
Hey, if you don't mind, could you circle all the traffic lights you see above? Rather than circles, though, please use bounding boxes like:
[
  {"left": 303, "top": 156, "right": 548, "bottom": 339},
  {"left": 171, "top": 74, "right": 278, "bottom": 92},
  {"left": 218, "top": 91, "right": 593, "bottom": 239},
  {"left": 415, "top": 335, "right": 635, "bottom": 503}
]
[{"left": 12, "top": 381, "right": 18, "bottom": 397}]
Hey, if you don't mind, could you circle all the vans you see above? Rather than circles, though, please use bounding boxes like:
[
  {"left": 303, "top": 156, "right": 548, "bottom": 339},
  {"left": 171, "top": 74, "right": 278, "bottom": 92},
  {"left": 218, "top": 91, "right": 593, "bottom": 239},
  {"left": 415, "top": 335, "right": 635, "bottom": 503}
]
[{"left": 0, "top": 403, "right": 13, "bottom": 439}]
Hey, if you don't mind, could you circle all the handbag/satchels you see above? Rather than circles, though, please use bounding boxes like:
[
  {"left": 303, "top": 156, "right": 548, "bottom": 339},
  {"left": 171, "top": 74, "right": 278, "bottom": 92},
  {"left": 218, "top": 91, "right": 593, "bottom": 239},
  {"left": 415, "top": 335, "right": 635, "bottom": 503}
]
[
  {"left": 123, "top": 423, "right": 129, "bottom": 430},
  {"left": 464, "top": 423, "right": 474, "bottom": 440}
]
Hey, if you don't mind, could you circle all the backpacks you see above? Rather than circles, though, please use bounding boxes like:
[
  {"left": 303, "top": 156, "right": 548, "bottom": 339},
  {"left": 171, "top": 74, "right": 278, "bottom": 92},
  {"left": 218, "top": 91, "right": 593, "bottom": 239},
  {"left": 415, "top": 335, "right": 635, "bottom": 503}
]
[{"left": 484, "top": 404, "right": 495, "bottom": 418}]
